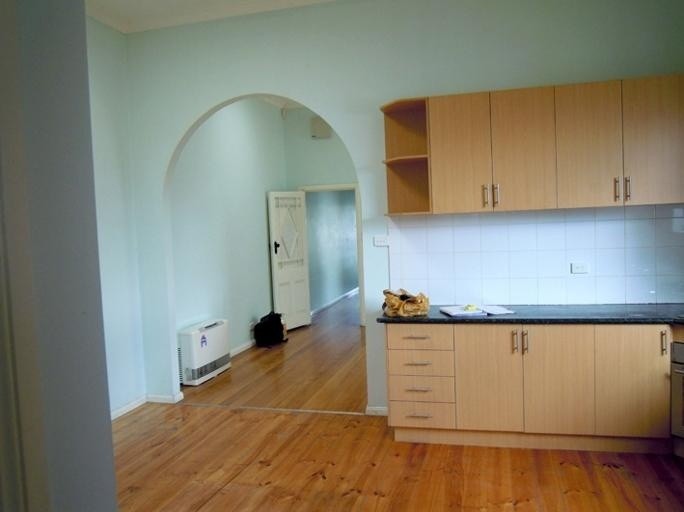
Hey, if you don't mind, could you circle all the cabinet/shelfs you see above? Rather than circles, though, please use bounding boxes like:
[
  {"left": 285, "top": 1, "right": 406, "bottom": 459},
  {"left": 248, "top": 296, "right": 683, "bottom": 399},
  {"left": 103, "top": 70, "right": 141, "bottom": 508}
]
[
  {"left": 379, "top": 97, "right": 431, "bottom": 219},
  {"left": 455, "top": 316, "right": 594, "bottom": 451},
  {"left": 425, "top": 86, "right": 555, "bottom": 215},
  {"left": 376, "top": 318, "right": 456, "bottom": 446},
  {"left": 594, "top": 317, "right": 672, "bottom": 454},
  {"left": 554, "top": 75, "right": 683, "bottom": 208}
]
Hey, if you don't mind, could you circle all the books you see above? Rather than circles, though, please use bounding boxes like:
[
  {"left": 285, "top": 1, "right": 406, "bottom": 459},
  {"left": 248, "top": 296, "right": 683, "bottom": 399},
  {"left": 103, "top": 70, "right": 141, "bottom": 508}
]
[{"left": 440, "top": 305, "right": 514, "bottom": 317}]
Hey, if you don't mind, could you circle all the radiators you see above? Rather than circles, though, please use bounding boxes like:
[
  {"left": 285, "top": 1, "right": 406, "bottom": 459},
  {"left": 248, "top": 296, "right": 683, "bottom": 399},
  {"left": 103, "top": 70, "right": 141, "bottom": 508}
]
[{"left": 178, "top": 319, "right": 232, "bottom": 387}]
